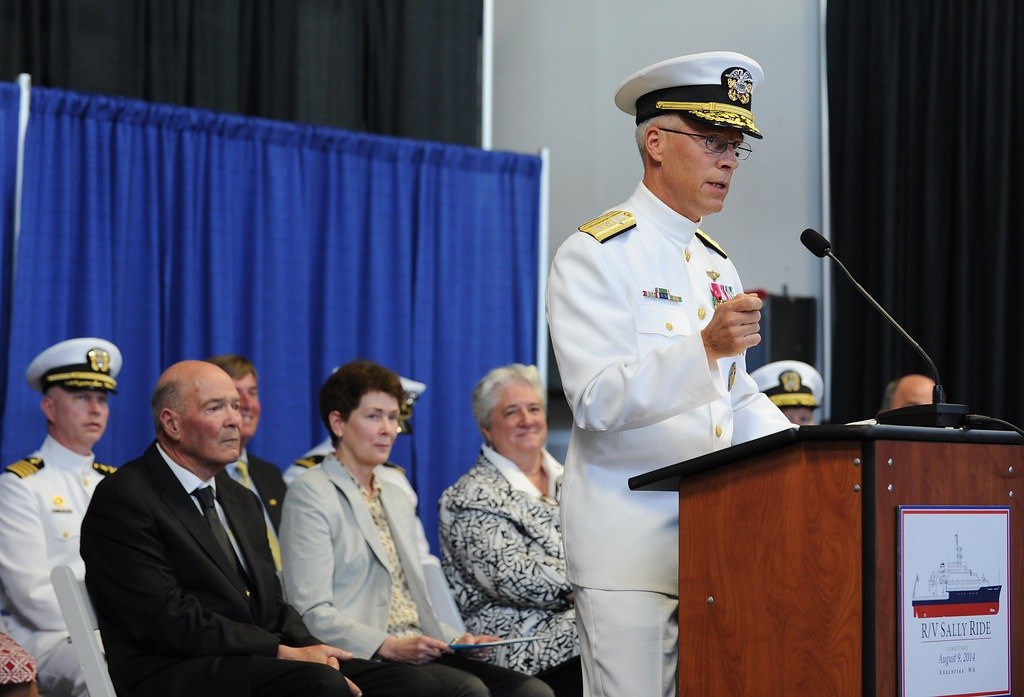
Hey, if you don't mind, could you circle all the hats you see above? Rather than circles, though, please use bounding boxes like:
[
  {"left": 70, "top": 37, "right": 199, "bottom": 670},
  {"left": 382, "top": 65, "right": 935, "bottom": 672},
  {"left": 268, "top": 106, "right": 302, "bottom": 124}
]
[
  {"left": 748, "top": 360, "right": 824, "bottom": 409},
  {"left": 614, "top": 51, "right": 764, "bottom": 140},
  {"left": 26, "top": 337, "right": 123, "bottom": 396},
  {"left": 333, "top": 366, "right": 426, "bottom": 436}
]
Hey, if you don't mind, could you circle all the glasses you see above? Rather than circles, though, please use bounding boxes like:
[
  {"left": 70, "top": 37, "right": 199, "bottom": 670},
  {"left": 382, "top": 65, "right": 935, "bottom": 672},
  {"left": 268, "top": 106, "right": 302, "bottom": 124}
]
[{"left": 658, "top": 128, "right": 753, "bottom": 160}]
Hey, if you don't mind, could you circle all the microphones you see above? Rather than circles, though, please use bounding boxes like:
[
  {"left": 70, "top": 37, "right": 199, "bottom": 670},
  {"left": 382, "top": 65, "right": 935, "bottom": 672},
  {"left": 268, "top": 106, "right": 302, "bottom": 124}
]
[{"left": 800, "top": 228, "right": 969, "bottom": 429}]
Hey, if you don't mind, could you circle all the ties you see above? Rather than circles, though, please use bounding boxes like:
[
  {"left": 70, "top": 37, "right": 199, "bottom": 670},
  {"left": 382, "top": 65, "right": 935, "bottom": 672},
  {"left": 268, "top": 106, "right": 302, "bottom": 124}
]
[
  {"left": 191, "top": 485, "right": 255, "bottom": 592},
  {"left": 235, "top": 460, "right": 282, "bottom": 571}
]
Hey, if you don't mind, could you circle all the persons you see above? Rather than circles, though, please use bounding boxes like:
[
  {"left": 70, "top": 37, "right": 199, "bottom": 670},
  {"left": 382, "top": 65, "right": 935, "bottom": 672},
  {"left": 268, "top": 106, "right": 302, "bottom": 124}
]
[
  {"left": 281, "top": 376, "right": 425, "bottom": 486},
  {"left": 0, "top": 338, "right": 122, "bottom": 697},
  {"left": 879, "top": 374, "right": 934, "bottom": 412},
  {"left": 751, "top": 361, "right": 825, "bottom": 426},
  {"left": 208, "top": 355, "right": 288, "bottom": 585},
  {"left": 280, "top": 361, "right": 555, "bottom": 697},
  {"left": 545, "top": 51, "right": 802, "bottom": 697},
  {"left": 79, "top": 360, "right": 437, "bottom": 697},
  {"left": 437, "top": 364, "right": 584, "bottom": 697}
]
